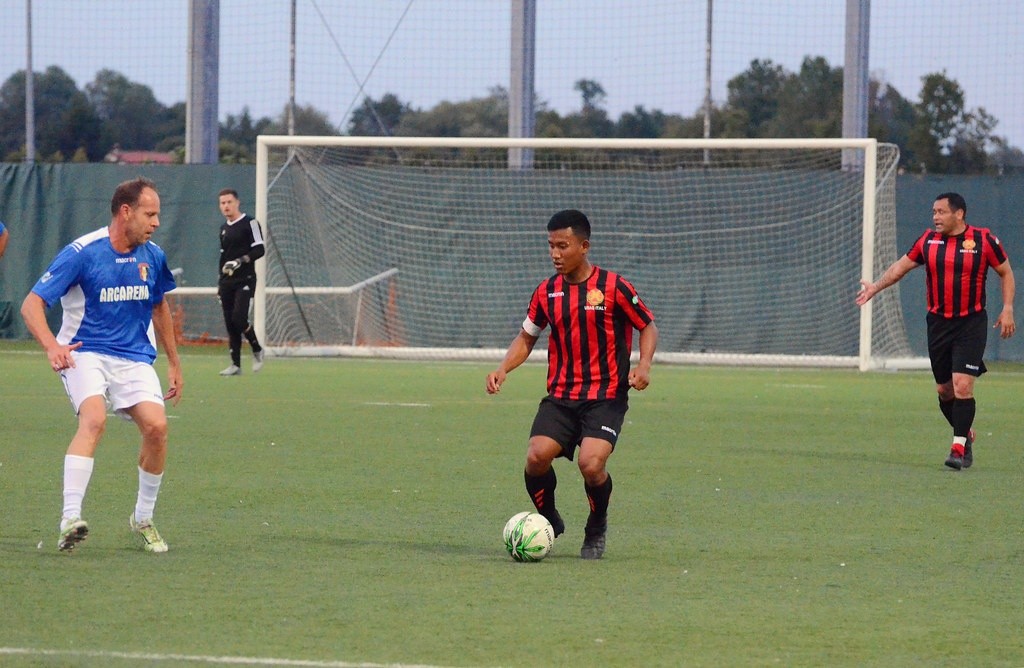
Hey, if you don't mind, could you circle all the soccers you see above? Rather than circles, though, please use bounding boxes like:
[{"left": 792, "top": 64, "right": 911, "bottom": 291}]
[{"left": 502, "top": 511, "right": 554, "bottom": 562}]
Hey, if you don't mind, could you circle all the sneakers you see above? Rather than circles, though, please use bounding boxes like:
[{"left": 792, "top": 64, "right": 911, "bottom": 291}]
[
  {"left": 252, "top": 346, "right": 265, "bottom": 371},
  {"left": 219, "top": 364, "right": 242, "bottom": 376},
  {"left": 581, "top": 511, "right": 608, "bottom": 560},
  {"left": 130, "top": 509, "right": 168, "bottom": 553},
  {"left": 58, "top": 518, "right": 90, "bottom": 552},
  {"left": 945, "top": 443, "right": 965, "bottom": 470},
  {"left": 546, "top": 510, "right": 565, "bottom": 539},
  {"left": 962, "top": 428, "right": 977, "bottom": 467}
]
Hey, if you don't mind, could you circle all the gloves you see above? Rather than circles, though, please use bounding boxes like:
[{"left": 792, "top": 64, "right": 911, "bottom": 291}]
[{"left": 222, "top": 256, "right": 247, "bottom": 276}]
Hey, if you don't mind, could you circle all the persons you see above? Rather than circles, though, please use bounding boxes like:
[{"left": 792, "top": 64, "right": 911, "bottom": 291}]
[
  {"left": 0, "top": 220, "right": 9, "bottom": 257},
  {"left": 20, "top": 177, "right": 183, "bottom": 553},
  {"left": 855, "top": 192, "right": 1017, "bottom": 470},
  {"left": 485, "top": 210, "right": 660, "bottom": 559},
  {"left": 219, "top": 188, "right": 266, "bottom": 376}
]
